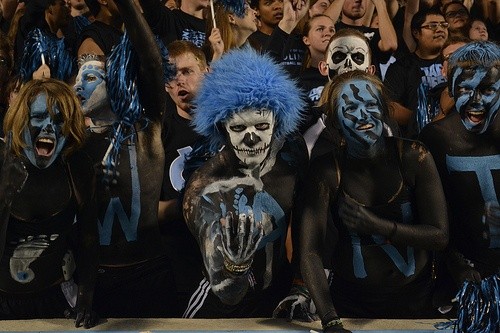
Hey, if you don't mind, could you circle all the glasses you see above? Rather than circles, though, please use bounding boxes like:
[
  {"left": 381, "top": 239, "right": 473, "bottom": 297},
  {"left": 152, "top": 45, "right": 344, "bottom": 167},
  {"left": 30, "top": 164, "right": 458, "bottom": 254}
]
[
  {"left": 420, "top": 21, "right": 450, "bottom": 31},
  {"left": 445, "top": 9, "right": 467, "bottom": 18}
]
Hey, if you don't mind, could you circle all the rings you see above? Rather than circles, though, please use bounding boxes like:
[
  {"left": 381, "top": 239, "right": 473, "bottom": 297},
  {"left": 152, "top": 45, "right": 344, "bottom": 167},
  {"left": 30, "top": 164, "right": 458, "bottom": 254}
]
[{"left": 353, "top": 204, "right": 359, "bottom": 210}]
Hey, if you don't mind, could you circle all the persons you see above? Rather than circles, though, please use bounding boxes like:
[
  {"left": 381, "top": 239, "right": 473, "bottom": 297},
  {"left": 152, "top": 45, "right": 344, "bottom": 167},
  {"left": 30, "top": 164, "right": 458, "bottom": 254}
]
[
  {"left": 184, "top": 41, "right": 318, "bottom": 322},
  {"left": 0, "top": 0, "right": 500, "bottom": 316},
  {"left": 74, "top": 0, "right": 178, "bottom": 319},
  {"left": 417, "top": 40, "right": 500, "bottom": 319},
  {"left": 301, "top": 71, "right": 448, "bottom": 333},
  {"left": 0, "top": 79, "right": 101, "bottom": 328}
]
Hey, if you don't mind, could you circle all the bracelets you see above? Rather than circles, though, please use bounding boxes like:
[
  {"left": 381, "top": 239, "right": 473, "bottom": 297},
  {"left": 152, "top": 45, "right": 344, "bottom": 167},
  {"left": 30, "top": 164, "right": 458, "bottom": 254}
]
[
  {"left": 384, "top": 221, "right": 398, "bottom": 240},
  {"left": 223, "top": 259, "right": 254, "bottom": 273},
  {"left": 320, "top": 309, "right": 343, "bottom": 329}
]
